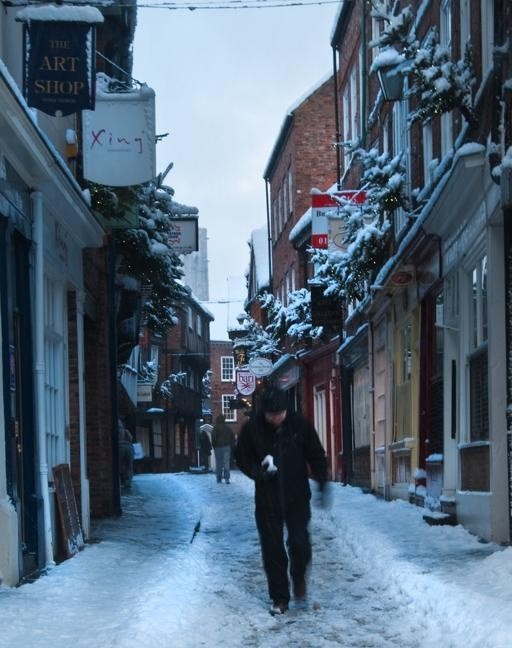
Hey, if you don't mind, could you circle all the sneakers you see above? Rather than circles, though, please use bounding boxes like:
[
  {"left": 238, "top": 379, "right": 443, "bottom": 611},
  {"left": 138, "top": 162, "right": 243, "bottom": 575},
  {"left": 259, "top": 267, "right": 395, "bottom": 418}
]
[{"left": 270, "top": 599, "right": 289, "bottom": 614}]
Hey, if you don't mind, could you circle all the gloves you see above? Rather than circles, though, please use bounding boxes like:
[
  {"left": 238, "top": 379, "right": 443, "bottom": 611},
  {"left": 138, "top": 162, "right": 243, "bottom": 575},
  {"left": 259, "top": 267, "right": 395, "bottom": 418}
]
[{"left": 253, "top": 463, "right": 273, "bottom": 481}]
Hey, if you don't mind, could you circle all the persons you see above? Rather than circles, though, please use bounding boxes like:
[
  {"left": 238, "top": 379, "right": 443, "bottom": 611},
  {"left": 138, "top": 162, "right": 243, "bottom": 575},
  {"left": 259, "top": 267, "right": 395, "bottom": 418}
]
[
  {"left": 210, "top": 414, "right": 236, "bottom": 484},
  {"left": 201, "top": 430, "right": 212, "bottom": 472},
  {"left": 237, "top": 387, "right": 327, "bottom": 617}
]
